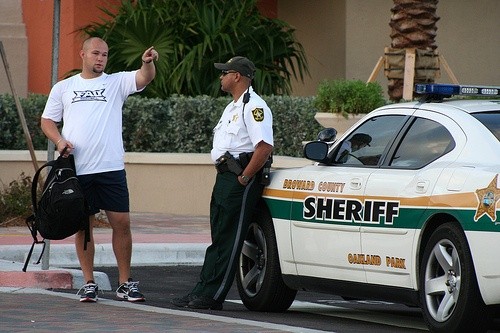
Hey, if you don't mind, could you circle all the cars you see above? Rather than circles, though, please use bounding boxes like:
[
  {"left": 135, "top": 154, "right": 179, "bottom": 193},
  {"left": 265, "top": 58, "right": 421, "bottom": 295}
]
[{"left": 236, "top": 83, "right": 500, "bottom": 333}]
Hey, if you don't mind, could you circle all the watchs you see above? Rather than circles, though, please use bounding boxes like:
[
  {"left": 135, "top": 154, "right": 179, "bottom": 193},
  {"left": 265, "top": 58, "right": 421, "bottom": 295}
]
[{"left": 241, "top": 175, "right": 249, "bottom": 183}]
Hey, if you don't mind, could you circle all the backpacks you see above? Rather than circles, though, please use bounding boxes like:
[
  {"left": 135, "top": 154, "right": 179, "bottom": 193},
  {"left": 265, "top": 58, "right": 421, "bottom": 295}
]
[{"left": 22, "top": 146, "right": 100, "bottom": 272}]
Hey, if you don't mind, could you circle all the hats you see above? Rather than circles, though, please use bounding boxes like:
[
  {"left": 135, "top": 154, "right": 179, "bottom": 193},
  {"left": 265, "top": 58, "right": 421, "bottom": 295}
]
[
  {"left": 213, "top": 56, "right": 257, "bottom": 80},
  {"left": 347, "top": 133, "right": 372, "bottom": 147}
]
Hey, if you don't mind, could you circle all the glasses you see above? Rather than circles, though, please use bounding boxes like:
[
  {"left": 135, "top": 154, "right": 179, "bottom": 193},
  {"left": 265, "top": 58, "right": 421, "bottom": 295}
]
[{"left": 221, "top": 70, "right": 237, "bottom": 76}]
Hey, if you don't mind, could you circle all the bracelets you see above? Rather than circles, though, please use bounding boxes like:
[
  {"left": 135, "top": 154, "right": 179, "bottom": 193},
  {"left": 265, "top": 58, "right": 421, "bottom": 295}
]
[
  {"left": 143, "top": 60, "right": 152, "bottom": 64},
  {"left": 56, "top": 139, "right": 62, "bottom": 145}
]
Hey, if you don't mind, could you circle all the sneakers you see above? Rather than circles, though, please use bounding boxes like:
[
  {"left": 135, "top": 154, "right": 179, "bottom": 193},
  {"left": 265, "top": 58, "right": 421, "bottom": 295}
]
[
  {"left": 115, "top": 277, "right": 147, "bottom": 302},
  {"left": 188, "top": 296, "right": 224, "bottom": 311},
  {"left": 170, "top": 291, "right": 202, "bottom": 307},
  {"left": 75, "top": 280, "right": 104, "bottom": 302}
]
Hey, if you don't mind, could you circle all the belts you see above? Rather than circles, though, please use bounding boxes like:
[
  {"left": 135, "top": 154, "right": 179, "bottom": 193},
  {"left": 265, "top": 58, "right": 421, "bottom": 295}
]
[{"left": 215, "top": 163, "right": 230, "bottom": 173}]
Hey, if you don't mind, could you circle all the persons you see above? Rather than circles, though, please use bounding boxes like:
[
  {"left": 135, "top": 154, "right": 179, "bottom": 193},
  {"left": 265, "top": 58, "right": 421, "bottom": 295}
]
[
  {"left": 335, "top": 133, "right": 372, "bottom": 163},
  {"left": 169, "top": 56, "right": 274, "bottom": 312},
  {"left": 41, "top": 37, "right": 159, "bottom": 302}
]
